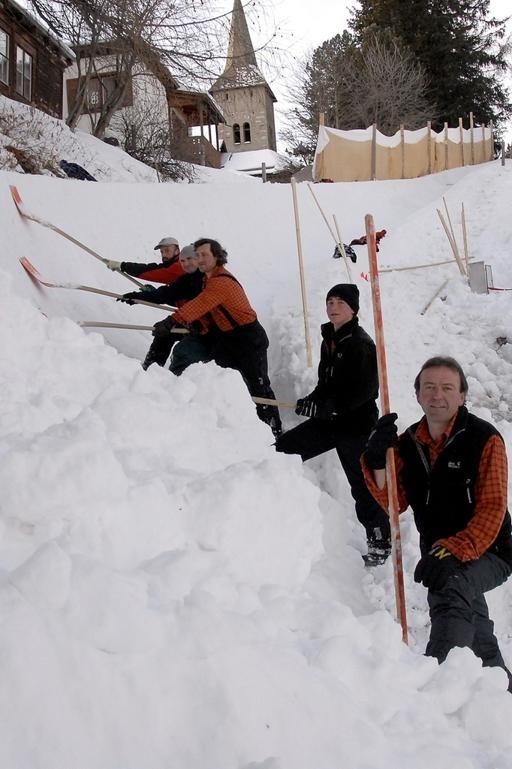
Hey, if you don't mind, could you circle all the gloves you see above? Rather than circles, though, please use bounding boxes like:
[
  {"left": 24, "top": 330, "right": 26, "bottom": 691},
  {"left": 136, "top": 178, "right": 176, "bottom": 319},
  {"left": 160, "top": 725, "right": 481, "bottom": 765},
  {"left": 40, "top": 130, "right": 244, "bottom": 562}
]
[
  {"left": 412, "top": 544, "right": 459, "bottom": 591},
  {"left": 295, "top": 391, "right": 326, "bottom": 422},
  {"left": 116, "top": 291, "right": 145, "bottom": 306},
  {"left": 102, "top": 259, "right": 124, "bottom": 273},
  {"left": 362, "top": 412, "right": 400, "bottom": 469},
  {"left": 151, "top": 315, "right": 175, "bottom": 337}
]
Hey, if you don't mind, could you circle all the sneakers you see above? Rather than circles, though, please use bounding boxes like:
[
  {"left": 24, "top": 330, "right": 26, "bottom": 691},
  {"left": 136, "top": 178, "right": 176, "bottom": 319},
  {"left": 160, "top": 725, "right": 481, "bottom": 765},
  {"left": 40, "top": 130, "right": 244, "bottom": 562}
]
[{"left": 362, "top": 539, "right": 392, "bottom": 566}]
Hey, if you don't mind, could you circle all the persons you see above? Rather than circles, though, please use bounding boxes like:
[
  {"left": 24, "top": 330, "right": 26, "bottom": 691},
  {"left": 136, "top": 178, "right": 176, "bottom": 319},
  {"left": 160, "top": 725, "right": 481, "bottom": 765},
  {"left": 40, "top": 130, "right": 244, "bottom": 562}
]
[
  {"left": 152, "top": 239, "right": 283, "bottom": 440},
  {"left": 116, "top": 244, "right": 207, "bottom": 370},
  {"left": 102, "top": 238, "right": 185, "bottom": 309},
  {"left": 359, "top": 357, "right": 512, "bottom": 694},
  {"left": 275, "top": 283, "right": 392, "bottom": 566}
]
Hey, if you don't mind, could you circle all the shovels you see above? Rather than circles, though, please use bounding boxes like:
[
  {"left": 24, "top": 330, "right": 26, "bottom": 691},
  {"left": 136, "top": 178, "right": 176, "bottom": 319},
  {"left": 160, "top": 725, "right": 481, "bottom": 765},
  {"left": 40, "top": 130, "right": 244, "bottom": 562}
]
[
  {"left": 9, "top": 184, "right": 147, "bottom": 290},
  {"left": 19, "top": 256, "right": 179, "bottom": 312}
]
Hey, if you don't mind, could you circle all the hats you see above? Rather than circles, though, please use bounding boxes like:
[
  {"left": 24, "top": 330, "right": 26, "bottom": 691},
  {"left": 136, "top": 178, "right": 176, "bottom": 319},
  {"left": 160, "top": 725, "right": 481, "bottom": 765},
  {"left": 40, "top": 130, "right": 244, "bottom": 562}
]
[
  {"left": 179, "top": 242, "right": 195, "bottom": 259},
  {"left": 154, "top": 235, "right": 180, "bottom": 251},
  {"left": 326, "top": 283, "right": 362, "bottom": 313}
]
